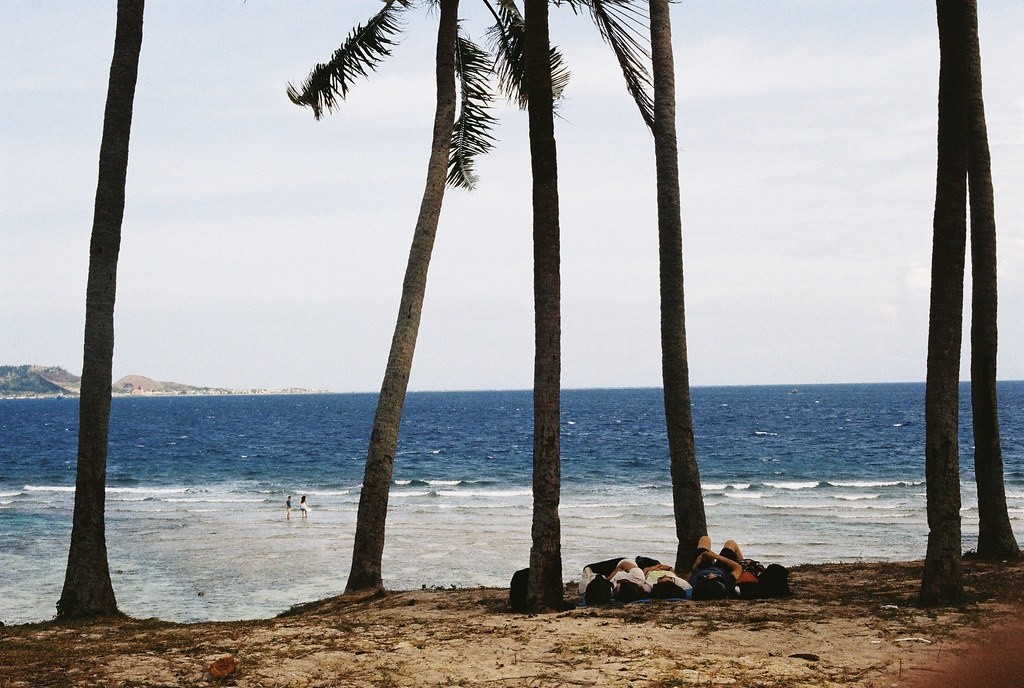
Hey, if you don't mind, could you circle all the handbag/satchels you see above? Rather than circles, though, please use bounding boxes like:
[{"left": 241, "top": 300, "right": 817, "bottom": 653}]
[{"left": 758, "top": 564, "right": 790, "bottom": 596}]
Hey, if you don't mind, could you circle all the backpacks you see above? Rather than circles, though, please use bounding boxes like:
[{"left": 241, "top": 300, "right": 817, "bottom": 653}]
[{"left": 510, "top": 568, "right": 529, "bottom": 611}]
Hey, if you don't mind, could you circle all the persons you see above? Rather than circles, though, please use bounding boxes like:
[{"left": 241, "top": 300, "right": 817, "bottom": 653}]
[
  {"left": 583, "top": 536, "right": 743, "bottom": 602},
  {"left": 285, "top": 496, "right": 291, "bottom": 520},
  {"left": 300, "top": 496, "right": 307, "bottom": 517}
]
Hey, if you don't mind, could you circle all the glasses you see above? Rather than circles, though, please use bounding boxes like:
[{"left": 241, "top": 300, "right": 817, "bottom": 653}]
[{"left": 658, "top": 577, "right": 675, "bottom": 584}]
